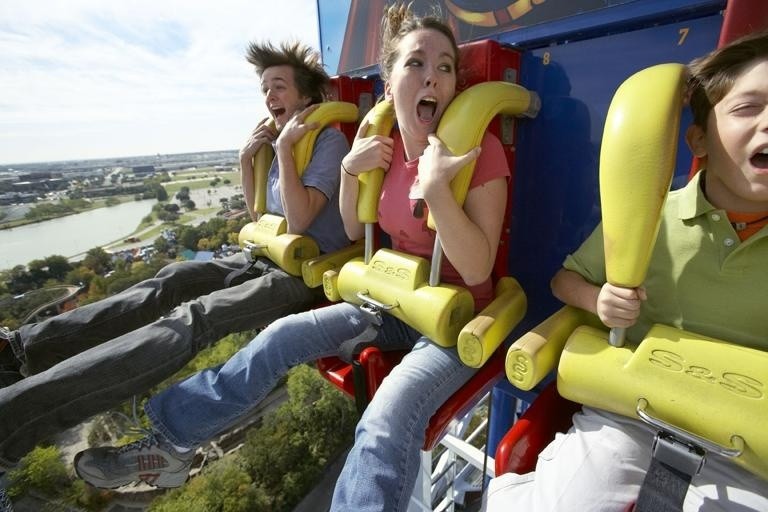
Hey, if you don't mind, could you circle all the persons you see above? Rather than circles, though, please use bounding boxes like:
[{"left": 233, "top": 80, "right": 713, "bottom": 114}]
[
  {"left": 0, "top": 41, "right": 354, "bottom": 479},
  {"left": 479, "top": 30, "right": 767, "bottom": 512},
  {"left": 73, "top": 0, "right": 512, "bottom": 512}
]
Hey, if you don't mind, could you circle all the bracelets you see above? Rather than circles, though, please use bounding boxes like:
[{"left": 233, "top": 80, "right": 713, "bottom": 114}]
[{"left": 340, "top": 161, "right": 359, "bottom": 177}]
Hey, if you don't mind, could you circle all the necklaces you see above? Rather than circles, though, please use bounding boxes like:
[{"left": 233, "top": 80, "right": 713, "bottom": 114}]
[{"left": 727, "top": 216, "right": 767, "bottom": 233}]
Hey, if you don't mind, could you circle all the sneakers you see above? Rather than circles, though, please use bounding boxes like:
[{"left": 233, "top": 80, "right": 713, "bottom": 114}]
[
  {"left": 0, "top": 326, "right": 30, "bottom": 387},
  {"left": 70, "top": 443, "right": 194, "bottom": 493}
]
